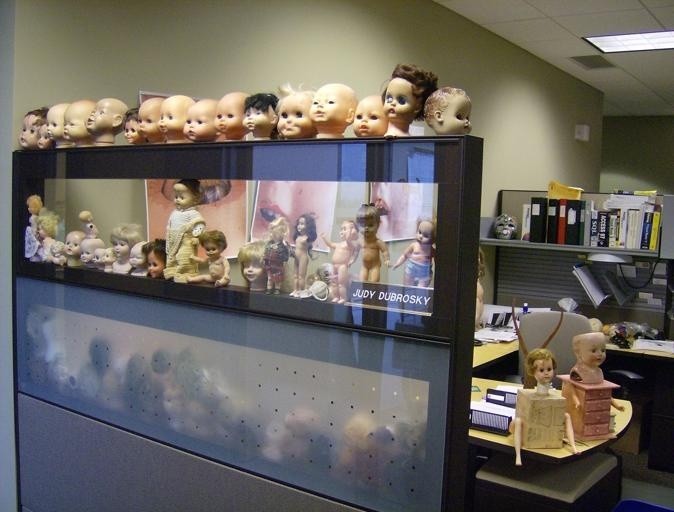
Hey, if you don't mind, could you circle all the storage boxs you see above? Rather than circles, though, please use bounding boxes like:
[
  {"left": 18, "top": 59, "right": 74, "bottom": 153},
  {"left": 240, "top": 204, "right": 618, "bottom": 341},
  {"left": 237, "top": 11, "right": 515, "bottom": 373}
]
[
  {"left": 613, "top": 497, "right": 673, "bottom": 512},
  {"left": 609, "top": 399, "right": 650, "bottom": 455},
  {"left": 474, "top": 452, "right": 623, "bottom": 512}
]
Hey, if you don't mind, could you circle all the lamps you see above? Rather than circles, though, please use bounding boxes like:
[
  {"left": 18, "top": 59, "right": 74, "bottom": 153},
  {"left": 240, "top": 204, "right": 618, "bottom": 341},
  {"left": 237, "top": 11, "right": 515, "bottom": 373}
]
[{"left": 587, "top": 254, "right": 664, "bottom": 289}]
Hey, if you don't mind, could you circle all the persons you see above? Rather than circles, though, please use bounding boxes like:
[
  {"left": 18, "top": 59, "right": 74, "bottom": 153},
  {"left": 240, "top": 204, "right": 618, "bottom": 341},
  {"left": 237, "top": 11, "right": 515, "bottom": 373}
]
[
  {"left": 180, "top": 202, "right": 437, "bottom": 305},
  {"left": 513, "top": 346, "right": 582, "bottom": 466},
  {"left": 24, "top": 195, "right": 164, "bottom": 277},
  {"left": 20, "top": 98, "right": 129, "bottom": 150},
  {"left": 164, "top": 178, "right": 205, "bottom": 280},
  {"left": 568, "top": 331, "right": 627, "bottom": 412},
  {"left": 122, "top": 90, "right": 279, "bottom": 145},
  {"left": 275, "top": 62, "right": 473, "bottom": 141},
  {"left": 12, "top": 60, "right": 475, "bottom": 512}
]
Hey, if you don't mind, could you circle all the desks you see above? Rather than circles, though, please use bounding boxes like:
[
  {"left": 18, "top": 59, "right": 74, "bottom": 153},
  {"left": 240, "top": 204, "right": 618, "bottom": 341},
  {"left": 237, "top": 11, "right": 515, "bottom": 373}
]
[{"left": 468, "top": 336, "right": 674, "bottom": 472}]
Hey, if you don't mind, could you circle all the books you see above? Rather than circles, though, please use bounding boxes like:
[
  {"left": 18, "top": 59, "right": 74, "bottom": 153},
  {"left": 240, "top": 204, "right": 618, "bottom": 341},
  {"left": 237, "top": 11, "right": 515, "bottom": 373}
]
[
  {"left": 517, "top": 181, "right": 663, "bottom": 255},
  {"left": 572, "top": 263, "right": 610, "bottom": 309}
]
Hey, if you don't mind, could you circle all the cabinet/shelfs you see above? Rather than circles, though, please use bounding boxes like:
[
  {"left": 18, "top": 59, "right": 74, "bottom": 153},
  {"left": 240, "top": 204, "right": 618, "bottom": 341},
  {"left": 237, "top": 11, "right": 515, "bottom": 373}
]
[{"left": 10, "top": 133, "right": 485, "bottom": 511}]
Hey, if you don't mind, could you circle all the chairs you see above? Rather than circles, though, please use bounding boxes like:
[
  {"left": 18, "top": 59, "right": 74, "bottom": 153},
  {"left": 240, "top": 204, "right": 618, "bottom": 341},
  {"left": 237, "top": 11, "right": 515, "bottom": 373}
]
[{"left": 507, "top": 311, "right": 644, "bottom": 401}]
